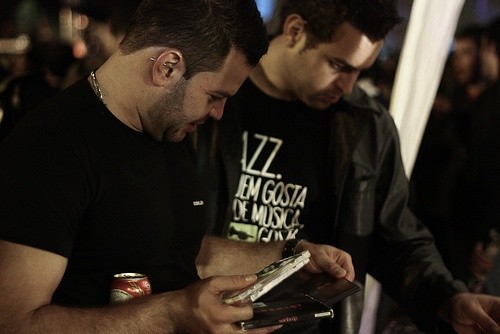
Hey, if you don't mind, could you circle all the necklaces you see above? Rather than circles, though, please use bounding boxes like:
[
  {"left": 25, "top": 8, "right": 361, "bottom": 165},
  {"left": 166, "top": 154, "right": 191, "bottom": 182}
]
[{"left": 91, "top": 70, "right": 108, "bottom": 109}]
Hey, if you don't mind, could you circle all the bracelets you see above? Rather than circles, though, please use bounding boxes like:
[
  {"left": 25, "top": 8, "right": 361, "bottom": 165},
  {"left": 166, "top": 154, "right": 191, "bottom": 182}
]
[{"left": 282, "top": 238, "right": 307, "bottom": 287}]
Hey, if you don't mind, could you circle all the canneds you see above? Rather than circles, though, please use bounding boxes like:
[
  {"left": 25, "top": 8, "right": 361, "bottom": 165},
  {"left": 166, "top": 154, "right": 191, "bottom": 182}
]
[{"left": 110, "top": 272, "right": 152, "bottom": 304}]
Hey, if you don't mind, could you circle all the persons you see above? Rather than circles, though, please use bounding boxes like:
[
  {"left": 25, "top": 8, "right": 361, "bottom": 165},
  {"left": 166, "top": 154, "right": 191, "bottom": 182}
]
[
  {"left": 408, "top": 16, "right": 499, "bottom": 281},
  {"left": 193, "top": 0, "right": 500, "bottom": 334},
  {"left": 0, "top": 0, "right": 356, "bottom": 334}
]
